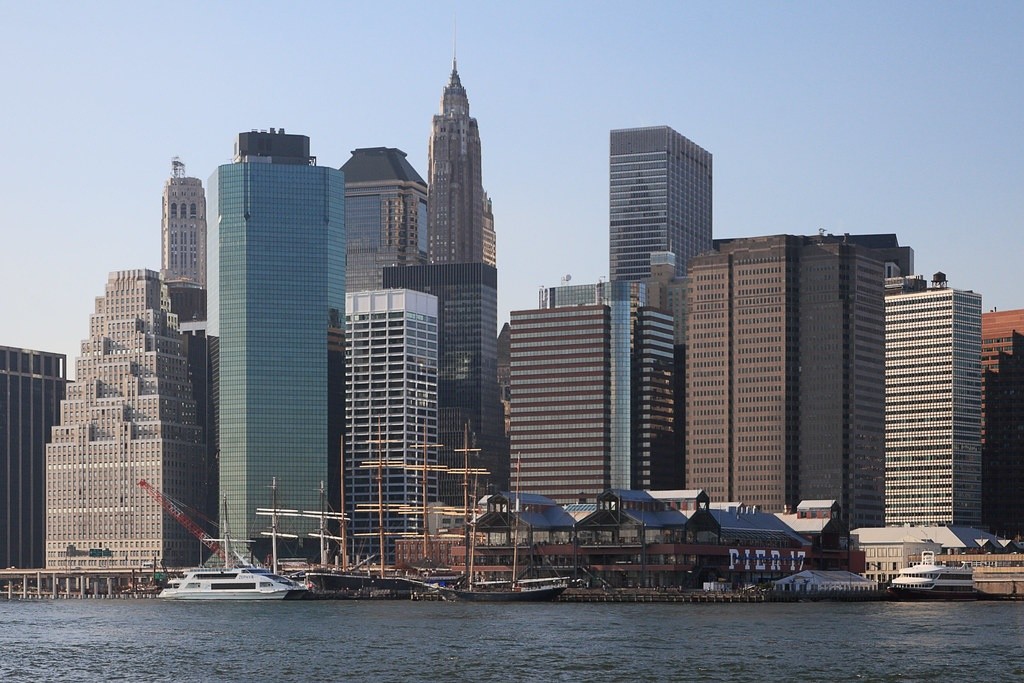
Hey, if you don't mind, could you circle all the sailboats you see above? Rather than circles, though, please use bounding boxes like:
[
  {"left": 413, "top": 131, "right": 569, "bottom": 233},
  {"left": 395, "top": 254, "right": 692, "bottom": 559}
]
[{"left": 258, "top": 420, "right": 571, "bottom": 608}]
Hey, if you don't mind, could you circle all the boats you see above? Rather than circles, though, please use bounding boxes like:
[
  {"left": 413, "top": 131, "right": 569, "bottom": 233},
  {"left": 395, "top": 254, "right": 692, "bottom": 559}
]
[
  {"left": 891, "top": 550, "right": 977, "bottom": 601},
  {"left": 157, "top": 490, "right": 311, "bottom": 602}
]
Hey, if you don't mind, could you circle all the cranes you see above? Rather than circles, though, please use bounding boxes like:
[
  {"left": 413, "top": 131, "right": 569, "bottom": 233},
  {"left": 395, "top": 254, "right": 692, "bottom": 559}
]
[{"left": 135, "top": 478, "right": 237, "bottom": 566}]
